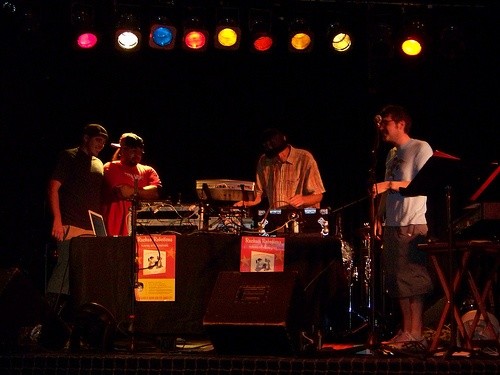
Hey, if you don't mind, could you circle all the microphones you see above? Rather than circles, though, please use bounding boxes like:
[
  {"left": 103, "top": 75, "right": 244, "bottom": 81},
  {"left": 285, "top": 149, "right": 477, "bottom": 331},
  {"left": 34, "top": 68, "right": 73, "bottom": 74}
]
[{"left": 374, "top": 114, "right": 382, "bottom": 131}]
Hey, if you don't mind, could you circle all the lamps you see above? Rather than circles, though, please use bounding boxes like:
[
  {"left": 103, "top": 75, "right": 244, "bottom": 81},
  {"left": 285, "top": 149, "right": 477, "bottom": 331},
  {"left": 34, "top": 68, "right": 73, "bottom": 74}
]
[
  {"left": 401, "top": 16, "right": 427, "bottom": 60},
  {"left": 148, "top": 12, "right": 178, "bottom": 51},
  {"left": 247, "top": 12, "right": 274, "bottom": 52},
  {"left": 286, "top": 17, "right": 312, "bottom": 53},
  {"left": 326, "top": 20, "right": 353, "bottom": 55},
  {"left": 182, "top": 14, "right": 210, "bottom": 53},
  {"left": 213, "top": 12, "right": 241, "bottom": 51},
  {"left": 75, "top": 9, "right": 101, "bottom": 51},
  {"left": 112, "top": 11, "right": 143, "bottom": 53}
]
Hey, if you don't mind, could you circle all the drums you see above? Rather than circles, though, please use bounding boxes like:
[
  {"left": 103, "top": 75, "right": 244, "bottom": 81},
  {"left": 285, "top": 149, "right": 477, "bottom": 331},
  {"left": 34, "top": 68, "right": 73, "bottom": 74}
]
[
  {"left": 359, "top": 218, "right": 375, "bottom": 255},
  {"left": 298, "top": 209, "right": 329, "bottom": 234},
  {"left": 256, "top": 209, "right": 292, "bottom": 234},
  {"left": 339, "top": 239, "right": 354, "bottom": 261},
  {"left": 219, "top": 206, "right": 248, "bottom": 219}
]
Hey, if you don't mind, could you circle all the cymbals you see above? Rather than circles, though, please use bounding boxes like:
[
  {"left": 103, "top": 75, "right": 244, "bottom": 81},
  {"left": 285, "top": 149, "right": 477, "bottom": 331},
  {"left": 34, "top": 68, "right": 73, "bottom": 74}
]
[{"left": 331, "top": 195, "right": 368, "bottom": 213}]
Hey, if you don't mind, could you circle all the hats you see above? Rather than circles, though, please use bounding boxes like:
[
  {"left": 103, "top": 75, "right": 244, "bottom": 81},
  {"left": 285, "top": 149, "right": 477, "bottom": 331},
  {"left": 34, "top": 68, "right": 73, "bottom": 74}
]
[
  {"left": 84, "top": 124, "right": 109, "bottom": 139},
  {"left": 262, "top": 134, "right": 288, "bottom": 159},
  {"left": 111, "top": 132, "right": 144, "bottom": 148}
]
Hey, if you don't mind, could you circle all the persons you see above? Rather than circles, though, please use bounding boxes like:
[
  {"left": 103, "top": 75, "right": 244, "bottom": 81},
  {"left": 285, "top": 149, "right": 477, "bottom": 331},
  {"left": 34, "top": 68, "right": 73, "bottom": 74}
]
[
  {"left": 46, "top": 124, "right": 108, "bottom": 294},
  {"left": 233, "top": 134, "right": 325, "bottom": 214},
  {"left": 371, "top": 104, "right": 434, "bottom": 348},
  {"left": 102, "top": 132, "right": 163, "bottom": 237}
]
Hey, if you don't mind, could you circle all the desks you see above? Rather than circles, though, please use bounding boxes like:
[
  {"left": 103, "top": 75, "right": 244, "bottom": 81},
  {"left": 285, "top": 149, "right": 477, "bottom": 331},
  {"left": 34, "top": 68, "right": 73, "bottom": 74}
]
[
  {"left": 417, "top": 238, "right": 500, "bottom": 353},
  {"left": 70, "top": 232, "right": 340, "bottom": 338}
]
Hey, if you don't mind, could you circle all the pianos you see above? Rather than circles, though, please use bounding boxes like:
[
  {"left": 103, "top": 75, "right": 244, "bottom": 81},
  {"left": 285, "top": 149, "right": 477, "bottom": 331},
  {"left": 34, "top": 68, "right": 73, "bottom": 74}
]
[{"left": 416, "top": 240, "right": 499, "bottom": 357}]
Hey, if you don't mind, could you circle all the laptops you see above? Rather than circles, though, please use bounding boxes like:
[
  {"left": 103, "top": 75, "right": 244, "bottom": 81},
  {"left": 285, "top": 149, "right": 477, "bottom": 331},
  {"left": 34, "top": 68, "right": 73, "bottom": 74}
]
[{"left": 88, "top": 209, "right": 119, "bottom": 237}]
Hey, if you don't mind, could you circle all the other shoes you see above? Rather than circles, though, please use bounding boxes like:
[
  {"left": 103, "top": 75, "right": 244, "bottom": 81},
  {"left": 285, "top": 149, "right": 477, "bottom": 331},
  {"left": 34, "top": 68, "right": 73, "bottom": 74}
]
[
  {"left": 381, "top": 329, "right": 404, "bottom": 345},
  {"left": 385, "top": 331, "right": 429, "bottom": 353}
]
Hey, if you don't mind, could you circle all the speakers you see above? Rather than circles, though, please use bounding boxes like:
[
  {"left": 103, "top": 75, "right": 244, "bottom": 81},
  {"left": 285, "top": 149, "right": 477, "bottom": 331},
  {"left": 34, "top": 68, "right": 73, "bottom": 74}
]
[
  {"left": 0, "top": 266, "right": 40, "bottom": 354},
  {"left": 202, "top": 271, "right": 321, "bottom": 356}
]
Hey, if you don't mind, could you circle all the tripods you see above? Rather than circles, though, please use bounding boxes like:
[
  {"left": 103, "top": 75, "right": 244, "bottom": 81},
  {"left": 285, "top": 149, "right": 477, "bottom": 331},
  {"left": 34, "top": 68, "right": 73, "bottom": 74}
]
[{"left": 328, "top": 282, "right": 370, "bottom": 341}]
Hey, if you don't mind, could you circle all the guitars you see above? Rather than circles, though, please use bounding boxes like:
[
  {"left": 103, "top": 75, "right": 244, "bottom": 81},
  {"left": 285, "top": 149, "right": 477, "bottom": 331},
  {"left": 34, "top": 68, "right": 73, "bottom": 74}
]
[{"left": 369, "top": 169, "right": 383, "bottom": 241}]
